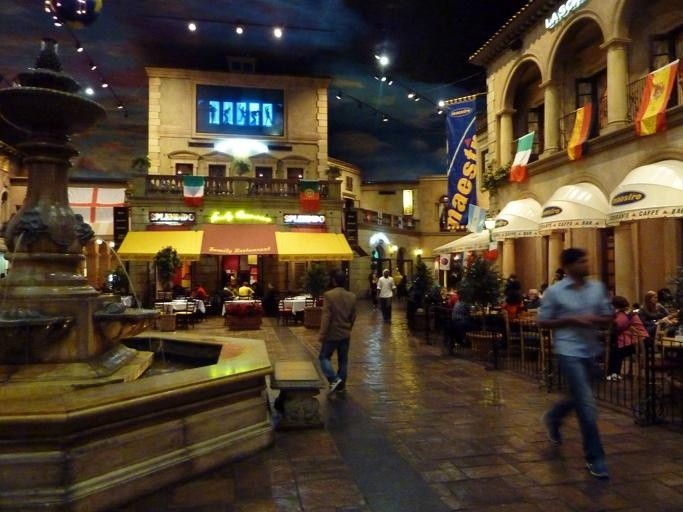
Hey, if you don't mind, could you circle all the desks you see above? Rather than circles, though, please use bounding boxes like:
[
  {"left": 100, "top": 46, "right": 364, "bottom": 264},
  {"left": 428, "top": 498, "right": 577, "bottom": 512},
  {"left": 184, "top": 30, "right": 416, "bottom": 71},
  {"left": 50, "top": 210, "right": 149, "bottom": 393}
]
[
  {"left": 222, "top": 300, "right": 263, "bottom": 326},
  {"left": 278, "top": 296, "right": 317, "bottom": 325},
  {"left": 155, "top": 302, "right": 197, "bottom": 328}
]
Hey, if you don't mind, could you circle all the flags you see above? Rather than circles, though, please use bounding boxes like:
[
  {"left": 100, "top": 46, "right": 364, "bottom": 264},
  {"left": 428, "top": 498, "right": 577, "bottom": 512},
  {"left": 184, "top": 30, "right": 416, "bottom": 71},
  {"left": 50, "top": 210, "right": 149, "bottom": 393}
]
[
  {"left": 634, "top": 57, "right": 681, "bottom": 137},
  {"left": 298, "top": 179, "right": 321, "bottom": 211},
  {"left": 507, "top": 131, "right": 535, "bottom": 182},
  {"left": 465, "top": 203, "right": 487, "bottom": 234},
  {"left": 182, "top": 175, "right": 205, "bottom": 207},
  {"left": 567, "top": 102, "right": 593, "bottom": 162}
]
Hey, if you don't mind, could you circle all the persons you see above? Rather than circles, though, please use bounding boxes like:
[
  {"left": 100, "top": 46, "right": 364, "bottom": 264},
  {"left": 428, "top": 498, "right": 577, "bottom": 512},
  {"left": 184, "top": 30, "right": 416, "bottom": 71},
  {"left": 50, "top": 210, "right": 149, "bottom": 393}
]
[
  {"left": 172, "top": 277, "right": 278, "bottom": 324},
  {"left": 318, "top": 270, "right": 358, "bottom": 395},
  {"left": 606, "top": 296, "right": 651, "bottom": 382},
  {"left": 376, "top": 269, "right": 396, "bottom": 324},
  {"left": 369, "top": 266, "right": 408, "bottom": 312},
  {"left": 431, "top": 267, "right": 566, "bottom": 321},
  {"left": 208, "top": 101, "right": 273, "bottom": 127},
  {"left": 539, "top": 246, "right": 616, "bottom": 477},
  {"left": 638, "top": 291, "right": 671, "bottom": 347}
]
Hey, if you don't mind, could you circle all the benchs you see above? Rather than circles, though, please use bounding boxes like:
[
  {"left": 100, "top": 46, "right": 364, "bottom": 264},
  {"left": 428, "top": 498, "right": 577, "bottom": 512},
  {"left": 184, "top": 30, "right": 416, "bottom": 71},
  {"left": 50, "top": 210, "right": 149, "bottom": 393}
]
[{"left": 269, "top": 360, "right": 327, "bottom": 431}]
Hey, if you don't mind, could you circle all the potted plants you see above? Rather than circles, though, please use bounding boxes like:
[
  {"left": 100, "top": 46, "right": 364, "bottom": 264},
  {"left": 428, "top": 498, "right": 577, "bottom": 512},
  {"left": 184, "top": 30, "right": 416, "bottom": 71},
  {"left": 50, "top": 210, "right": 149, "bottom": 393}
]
[
  {"left": 151, "top": 246, "right": 183, "bottom": 302},
  {"left": 299, "top": 262, "right": 331, "bottom": 328},
  {"left": 404, "top": 255, "right": 435, "bottom": 331},
  {"left": 456, "top": 254, "right": 510, "bottom": 361}
]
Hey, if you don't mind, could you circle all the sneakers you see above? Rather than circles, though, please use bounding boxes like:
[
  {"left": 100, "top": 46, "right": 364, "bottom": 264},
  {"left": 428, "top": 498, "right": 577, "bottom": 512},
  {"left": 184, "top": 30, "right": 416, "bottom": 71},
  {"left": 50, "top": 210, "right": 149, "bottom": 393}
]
[
  {"left": 606, "top": 373, "right": 622, "bottom": 380},
  {"left": 326, "top": 378, "right": 342, "bottom": 394},
  {"left": 585, "top": 462, "right": 609, "bottom": 477},
  {"left": 541, "top": 413, "right": 561, "bottom": 443}
]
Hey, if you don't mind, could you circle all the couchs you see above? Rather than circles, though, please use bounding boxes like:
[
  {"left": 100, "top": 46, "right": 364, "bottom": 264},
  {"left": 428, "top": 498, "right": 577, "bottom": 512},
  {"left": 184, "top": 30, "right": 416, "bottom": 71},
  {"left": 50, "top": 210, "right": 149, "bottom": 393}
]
[{"left": 225, "top": 303, "right": 264, "bottom": 330}]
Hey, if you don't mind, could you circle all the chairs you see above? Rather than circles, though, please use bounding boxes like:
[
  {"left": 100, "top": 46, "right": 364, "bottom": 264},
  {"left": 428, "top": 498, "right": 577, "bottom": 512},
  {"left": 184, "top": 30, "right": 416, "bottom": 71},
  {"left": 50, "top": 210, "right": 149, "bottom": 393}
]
[
  {"left": 276, "top": 296, "right": 289, "bottom": 326},
  {"left": 176, "top": 301, "right": 198, "bottom": 330},
  {"left": 296, "top": 297, "right": 315, "bottom": 323}
]
